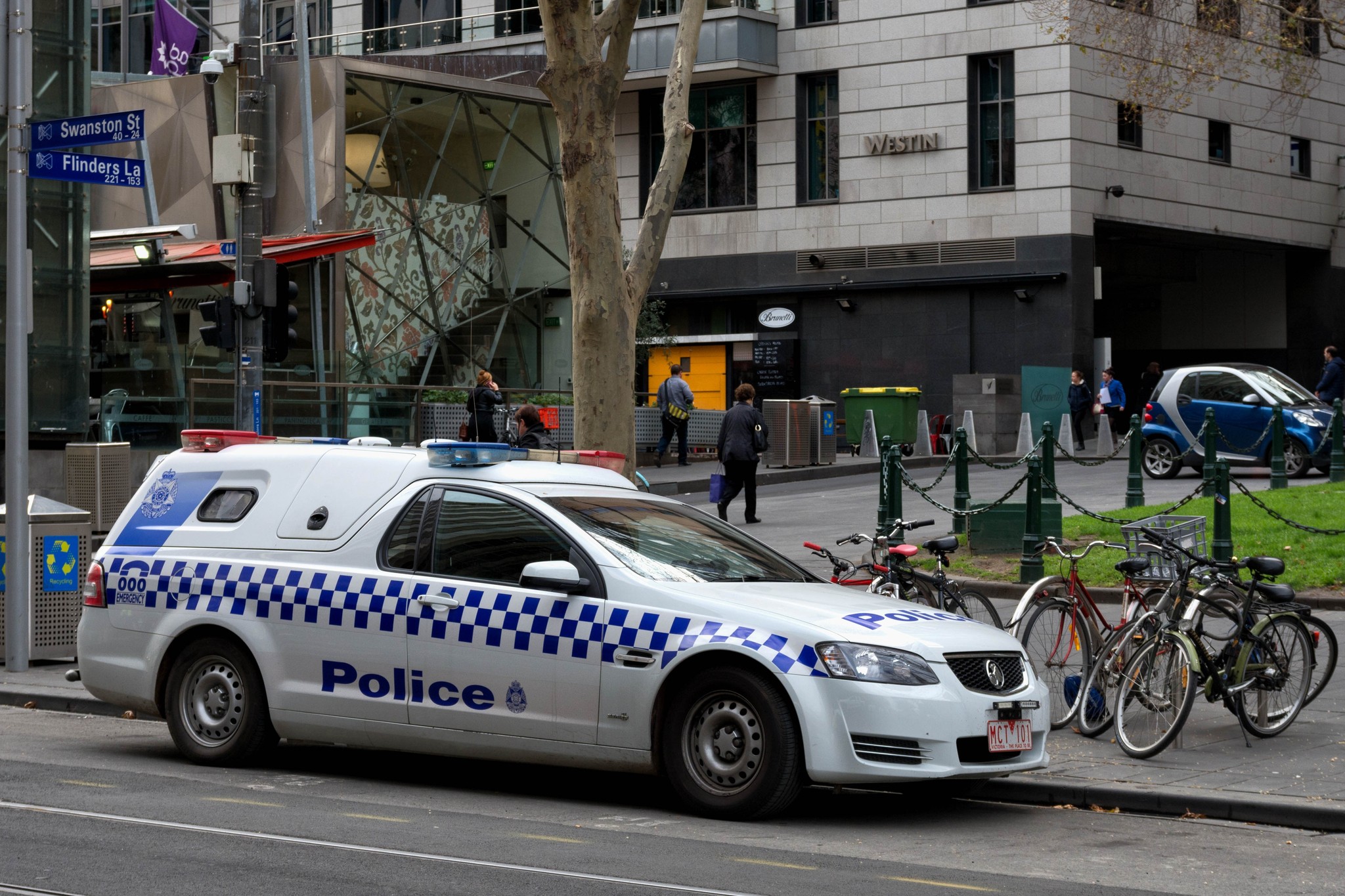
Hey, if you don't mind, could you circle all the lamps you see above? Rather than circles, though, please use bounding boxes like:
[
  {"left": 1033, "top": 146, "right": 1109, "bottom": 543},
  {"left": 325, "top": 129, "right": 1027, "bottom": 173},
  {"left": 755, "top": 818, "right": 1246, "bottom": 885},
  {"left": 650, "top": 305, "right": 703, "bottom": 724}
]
[
  {"left": 345, "top": 111, "right": 392, "bottom": 188},
  {"left": 132, "top": 243, "right": 154, "bottom": 265}
]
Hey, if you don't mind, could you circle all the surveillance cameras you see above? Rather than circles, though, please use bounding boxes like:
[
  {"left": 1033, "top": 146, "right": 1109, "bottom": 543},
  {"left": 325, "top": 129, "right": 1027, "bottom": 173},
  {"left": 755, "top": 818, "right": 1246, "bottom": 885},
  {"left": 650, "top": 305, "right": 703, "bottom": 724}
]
[{"left": 200, "top": 60, "right": 224, "bottom": 84}]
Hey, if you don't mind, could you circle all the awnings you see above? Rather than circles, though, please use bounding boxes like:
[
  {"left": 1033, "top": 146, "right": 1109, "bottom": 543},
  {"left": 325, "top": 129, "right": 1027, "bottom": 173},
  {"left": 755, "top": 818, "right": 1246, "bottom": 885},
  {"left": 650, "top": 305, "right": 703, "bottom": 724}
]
[{"left": 89, "top": 228, "right": 375, "bottom": 266}]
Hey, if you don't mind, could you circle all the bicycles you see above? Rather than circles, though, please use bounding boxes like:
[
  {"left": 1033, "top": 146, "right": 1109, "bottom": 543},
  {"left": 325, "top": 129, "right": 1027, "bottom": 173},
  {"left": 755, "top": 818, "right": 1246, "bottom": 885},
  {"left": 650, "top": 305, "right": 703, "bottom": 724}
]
[
  {"left": 493, "top": 407, "right": 552, "bottom": 448},
  {"left": 802, "top": 517, "right": 1339, "bottom": 738},
  {"left": 1113, "top": 524, "right": 1320, "bottom": 760}
]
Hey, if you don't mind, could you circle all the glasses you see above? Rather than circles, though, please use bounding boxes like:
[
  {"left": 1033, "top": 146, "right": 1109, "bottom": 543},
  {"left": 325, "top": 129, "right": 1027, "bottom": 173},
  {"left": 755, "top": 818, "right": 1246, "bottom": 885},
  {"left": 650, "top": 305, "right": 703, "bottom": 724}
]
[{"left": 1102, "top": 369, "right": 1107, "bottom": 374}]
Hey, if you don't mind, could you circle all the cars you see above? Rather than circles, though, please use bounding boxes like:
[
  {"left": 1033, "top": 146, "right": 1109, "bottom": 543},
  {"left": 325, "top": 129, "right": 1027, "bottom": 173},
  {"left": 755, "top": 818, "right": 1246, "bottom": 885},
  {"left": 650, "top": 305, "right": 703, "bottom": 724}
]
[
  {"left": 1140, "top": 362, "right": 1345, "bottom": 480},
  {"left": 63, "top": 428, "right": 1051, "bottom": 823}
]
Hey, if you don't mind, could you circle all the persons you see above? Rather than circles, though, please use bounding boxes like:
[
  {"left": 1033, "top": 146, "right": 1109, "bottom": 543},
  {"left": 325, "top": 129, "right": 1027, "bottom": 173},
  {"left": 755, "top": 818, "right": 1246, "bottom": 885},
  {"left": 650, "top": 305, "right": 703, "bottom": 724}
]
[
  {"left": 654, "top": 364, "right": 694, "bottom": 467},
  {"left": 1314, "top": 347, "right": 1343, "bottom": 404},
  {"left": 1096, "top": 367, "right": 1125, "bottom": 448},
  {"left": 467, "top": 370, "right": 503, "bottom": 442},
  {"left": 515, "top": 405, "right": 563, "bottom": 450},
  {"left": 718, "top": 384, "right": 768, "bottom": 523},
  {"left": 1068, "top": 372, "right": 1092, "bottom": 450},
  {"left": 1142, "top": 364, "right": 1160, "bottom": 393}
]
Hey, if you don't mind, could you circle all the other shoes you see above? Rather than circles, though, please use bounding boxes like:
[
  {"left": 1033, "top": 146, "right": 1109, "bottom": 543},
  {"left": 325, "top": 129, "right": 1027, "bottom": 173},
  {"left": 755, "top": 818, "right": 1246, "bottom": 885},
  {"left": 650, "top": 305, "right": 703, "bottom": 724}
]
[
  {"left": 653, "top": 449, "right": 661, "bottom": 468},
  {"left": 746, "top": 516, "right": 761, "bottom": 523},
  {"left": 679, "top": 461, "right": 692, "bottom": 466}
]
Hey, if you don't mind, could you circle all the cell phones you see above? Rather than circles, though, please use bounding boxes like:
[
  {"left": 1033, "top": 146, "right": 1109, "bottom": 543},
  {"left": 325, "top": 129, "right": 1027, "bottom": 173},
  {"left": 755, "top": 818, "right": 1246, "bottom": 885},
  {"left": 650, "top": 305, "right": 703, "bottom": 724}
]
[{"left": 487, "top": 381, "right": 493, "bottom": 388}]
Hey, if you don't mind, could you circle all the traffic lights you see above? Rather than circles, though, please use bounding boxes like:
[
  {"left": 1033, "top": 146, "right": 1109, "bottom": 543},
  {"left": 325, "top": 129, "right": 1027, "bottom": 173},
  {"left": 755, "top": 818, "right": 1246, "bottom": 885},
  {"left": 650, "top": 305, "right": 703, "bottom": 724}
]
[
  {"left": 260, "top": 265, "right": 300, "bottom": 364},
  {"left": 198, "top": 295, "right": 236, "bottom": 352},
  {"left": 131, "top": 244, "right": 157, "bottom": 267}
]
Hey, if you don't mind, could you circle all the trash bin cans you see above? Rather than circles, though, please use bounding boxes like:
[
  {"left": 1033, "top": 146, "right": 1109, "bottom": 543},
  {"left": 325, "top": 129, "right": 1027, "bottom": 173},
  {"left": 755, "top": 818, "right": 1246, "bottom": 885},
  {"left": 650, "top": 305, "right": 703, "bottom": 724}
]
[
  {"left": 799, "top": 395, "right": 838, "bottom": 466},
  {"left": 64, "top": 442, "right": 132, "bottom": 533},
  {"left": 840, "top": 386, "right": 922, "bottom": 458},
  {"left": 1, "top": 494, "right": 92, "bottom": 662},
  {"left": 762, "top": 399, "right": 812, "bottom": 468}
]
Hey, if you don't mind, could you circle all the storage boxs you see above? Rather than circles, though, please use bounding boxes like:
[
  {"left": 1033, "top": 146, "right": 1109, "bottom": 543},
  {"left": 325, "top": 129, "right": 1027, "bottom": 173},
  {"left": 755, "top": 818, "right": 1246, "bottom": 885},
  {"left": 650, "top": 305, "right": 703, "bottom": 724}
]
[
  {"left": 537, "top": 408, "right": 559, "bottom": 429},
  {"left": 1120, "top": 514, "right": 1207, "bottom": 582}
]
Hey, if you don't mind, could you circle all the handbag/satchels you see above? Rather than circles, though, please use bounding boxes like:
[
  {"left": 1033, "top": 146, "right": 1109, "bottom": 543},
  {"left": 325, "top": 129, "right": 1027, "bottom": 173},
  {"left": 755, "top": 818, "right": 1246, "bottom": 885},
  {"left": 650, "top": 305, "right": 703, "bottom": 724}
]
[
  {"left": 459, "top": 421, "right": 471, "bottom": 442},
  {"left": 1079, "top": 393, "right": 1095, "bottom": 421},
  {"left": 1116, "top": 410, "right": 1130, "bottom": 435},
  {"left": 709, "top": 461, "right": 726, "bottom": 503},
  {"left": 662, "top": 402, "right": 690, "bottom": 429},
  {"left": 752, "top": 408, "right": 770, "bottom": 453}
]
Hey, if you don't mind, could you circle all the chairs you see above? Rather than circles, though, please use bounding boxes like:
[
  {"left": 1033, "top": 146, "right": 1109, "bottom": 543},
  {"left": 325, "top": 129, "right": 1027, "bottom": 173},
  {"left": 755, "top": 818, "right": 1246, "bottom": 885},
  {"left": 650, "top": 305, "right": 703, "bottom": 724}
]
[
  {"left": 85, "top": 389, "right": 129, "bottom": 443},
  {"left": 929, "top": 414, "right": 946, "bottom": 455},
  {"left": 940, "top": 414, "right": 953, "bottom": 455}
]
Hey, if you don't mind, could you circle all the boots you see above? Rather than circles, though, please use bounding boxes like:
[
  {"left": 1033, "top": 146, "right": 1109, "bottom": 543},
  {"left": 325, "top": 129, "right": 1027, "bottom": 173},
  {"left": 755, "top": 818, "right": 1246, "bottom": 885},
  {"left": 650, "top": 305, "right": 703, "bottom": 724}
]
[{"left": 1075, "top": 441, "right": 1085, "bottom": 451}]
